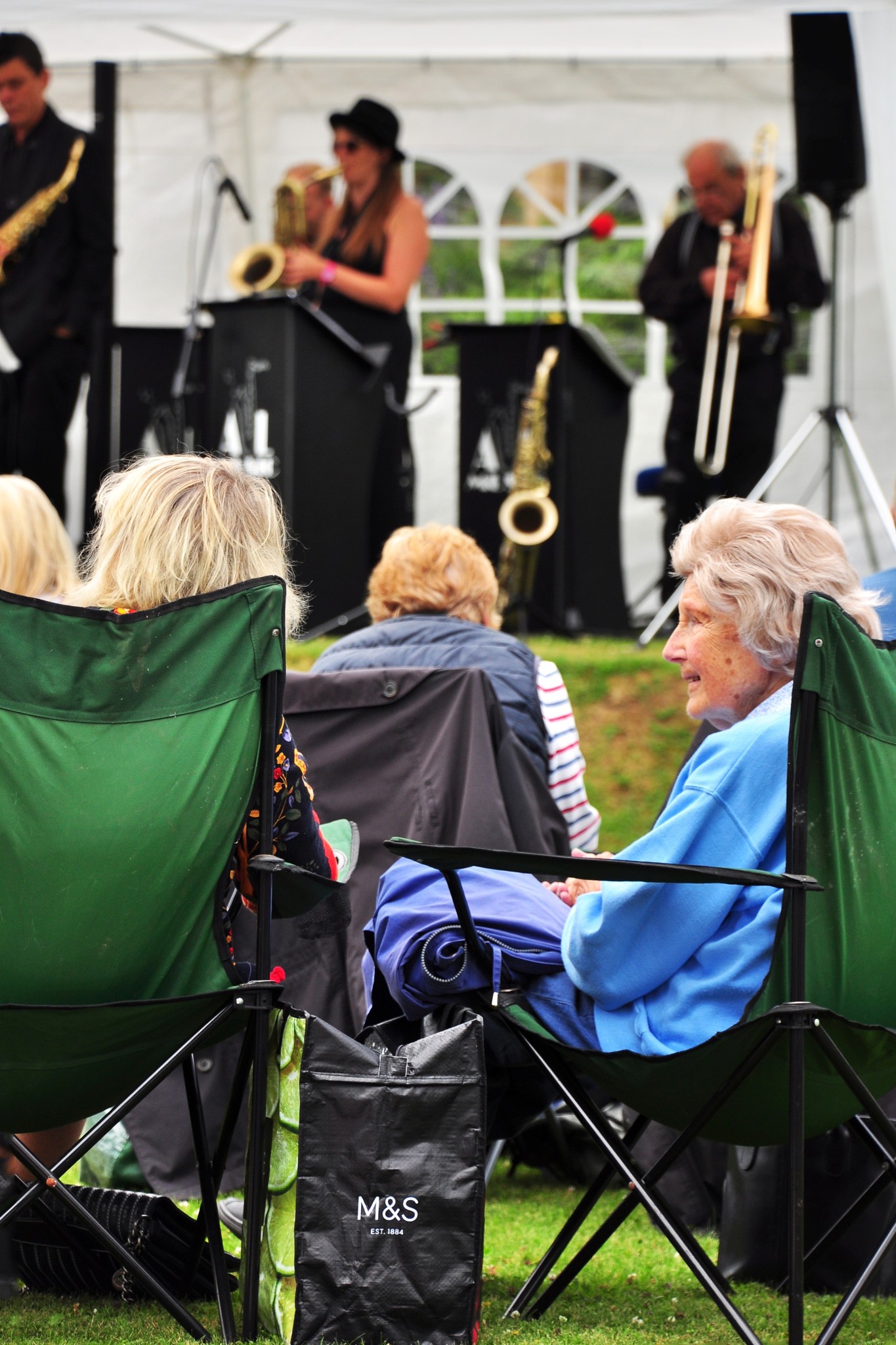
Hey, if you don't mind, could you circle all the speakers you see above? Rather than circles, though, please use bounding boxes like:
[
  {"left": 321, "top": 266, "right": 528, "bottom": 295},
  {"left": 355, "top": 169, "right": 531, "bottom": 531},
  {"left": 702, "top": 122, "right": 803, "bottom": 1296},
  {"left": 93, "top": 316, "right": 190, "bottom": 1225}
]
[{"left": 789, "top": 12, "right": 867, "bottom": 193}]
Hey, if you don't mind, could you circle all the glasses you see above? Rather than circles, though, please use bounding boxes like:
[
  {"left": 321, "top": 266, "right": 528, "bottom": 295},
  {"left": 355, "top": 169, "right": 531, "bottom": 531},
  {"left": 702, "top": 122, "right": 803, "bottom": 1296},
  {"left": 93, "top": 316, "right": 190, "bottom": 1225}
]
[{"left": 332, "top": 139, "right": 375, "bottom": 157}]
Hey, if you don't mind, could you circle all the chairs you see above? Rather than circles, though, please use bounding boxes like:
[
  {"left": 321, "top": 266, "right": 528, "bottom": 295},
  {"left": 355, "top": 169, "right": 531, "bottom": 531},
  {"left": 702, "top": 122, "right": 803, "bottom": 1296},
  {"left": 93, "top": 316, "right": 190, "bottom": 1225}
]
[
  {"left": 278, "top": 664, "right": 575, "bottom": 1188},
  {"left": 0, "top": 574, "right": 289, "bottom": 1345},
  {"left": 352, "top": 591, "right": 896, "bottom": 1345}
]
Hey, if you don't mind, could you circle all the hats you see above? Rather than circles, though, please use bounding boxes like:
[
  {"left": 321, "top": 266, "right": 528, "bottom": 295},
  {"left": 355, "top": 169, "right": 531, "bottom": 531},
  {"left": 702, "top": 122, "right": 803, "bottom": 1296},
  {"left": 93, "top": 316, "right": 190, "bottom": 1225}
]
[{"left": 328, "top": 99, "right": 405, "bottom": 167}]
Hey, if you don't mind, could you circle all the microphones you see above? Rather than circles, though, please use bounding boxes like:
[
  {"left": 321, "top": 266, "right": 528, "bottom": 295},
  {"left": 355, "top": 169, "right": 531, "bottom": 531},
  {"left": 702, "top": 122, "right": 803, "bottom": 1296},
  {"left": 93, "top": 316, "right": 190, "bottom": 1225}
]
[
  {"left": 212, "top": 155, "right": 250, "bottom": 222},
  {"left": 548, "top": 213, "right": 615, "bottom": 250}
]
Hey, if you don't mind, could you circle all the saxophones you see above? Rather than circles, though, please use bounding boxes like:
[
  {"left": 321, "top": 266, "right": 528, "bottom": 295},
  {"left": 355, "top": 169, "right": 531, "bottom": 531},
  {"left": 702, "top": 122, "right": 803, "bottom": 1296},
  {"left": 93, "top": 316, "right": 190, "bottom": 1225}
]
[
  {"left": 227, "top": 165, "right": 348, "bottom": 294},
  {"left": 491, "top": 345, "right": 560, "bottom": 631},
  {"left": 0, "top": 139, "right": 87, "bottom": 259}
]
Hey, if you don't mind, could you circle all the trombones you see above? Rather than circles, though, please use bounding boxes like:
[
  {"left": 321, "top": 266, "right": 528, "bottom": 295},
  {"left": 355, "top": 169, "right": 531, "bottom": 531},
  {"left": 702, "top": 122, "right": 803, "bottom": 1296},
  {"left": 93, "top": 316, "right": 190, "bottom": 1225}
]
[{"left": 693, "top": 123, "right": 785, "bottom": 475}]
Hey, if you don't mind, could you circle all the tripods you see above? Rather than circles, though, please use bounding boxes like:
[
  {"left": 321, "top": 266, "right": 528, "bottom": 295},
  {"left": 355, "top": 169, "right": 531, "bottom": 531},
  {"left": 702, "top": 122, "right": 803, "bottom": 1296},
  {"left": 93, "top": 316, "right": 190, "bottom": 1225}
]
[{"left": 637, "top": 190, "right": 896, "bottom": 647}]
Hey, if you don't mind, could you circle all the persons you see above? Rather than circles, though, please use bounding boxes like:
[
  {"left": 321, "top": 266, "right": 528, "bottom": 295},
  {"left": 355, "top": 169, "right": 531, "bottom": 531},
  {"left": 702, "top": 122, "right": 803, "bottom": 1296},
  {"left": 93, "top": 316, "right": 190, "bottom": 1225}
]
[
  {"left": 0, "top": 469, "right": 354, "bottom": 1184},
  {"left": 637, "top": 139, "right": 827, "bottom": 630},
  {"left": 0, "top": 29, "right": 102, "bottom": 530},
  {"left": 210, "top": 494, "right": 895, "bottom": 1245},
  {"left": 278, "top": 98, "right": 431, "bottom": 563},
  {"left": 305, "top": 521, "right": 602, "bottom": 853}
]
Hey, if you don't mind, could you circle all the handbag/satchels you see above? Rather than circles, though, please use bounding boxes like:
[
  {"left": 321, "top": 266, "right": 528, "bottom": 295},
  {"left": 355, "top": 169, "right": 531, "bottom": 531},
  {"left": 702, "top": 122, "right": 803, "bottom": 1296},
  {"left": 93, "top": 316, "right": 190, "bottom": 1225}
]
[
  {"left": 247, "top": 998, "right": 484, "bottom": 1343},
  {"left": 2, "top": 1175, "right": 239, "bottom": 1309},
  {"left": 717, "top": 1121, "right": 896, "bottom": 1302}
]
